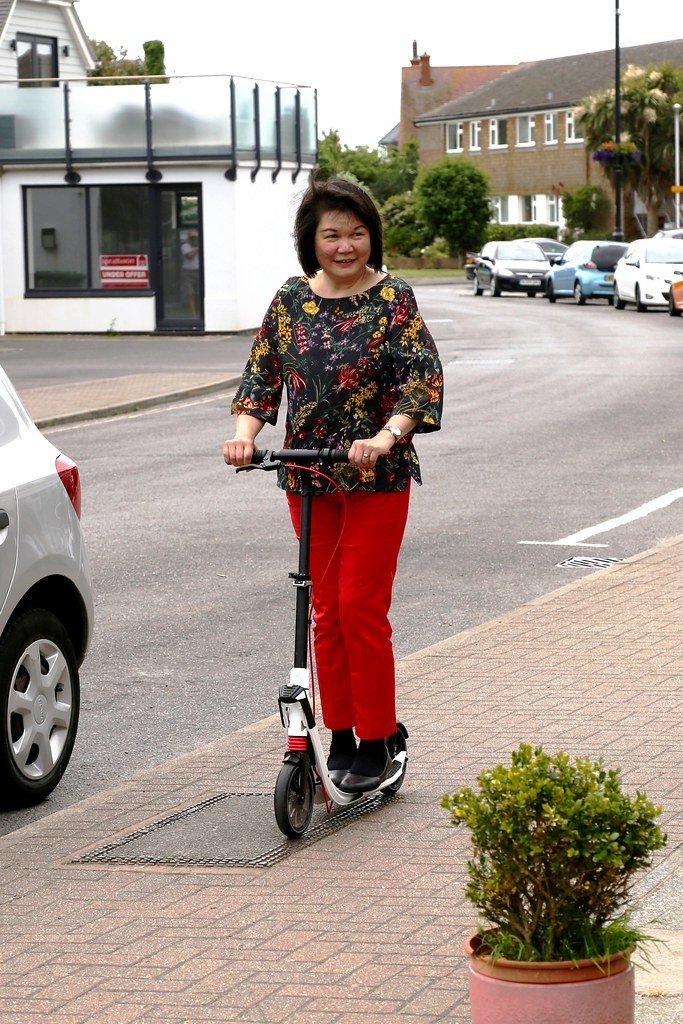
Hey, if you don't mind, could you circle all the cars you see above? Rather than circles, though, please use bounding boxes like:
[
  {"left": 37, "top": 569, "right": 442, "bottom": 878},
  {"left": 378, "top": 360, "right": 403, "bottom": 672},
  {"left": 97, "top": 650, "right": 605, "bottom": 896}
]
[
  {"left": 668, "top": 269, "right": 683, "bottom": 317},
  {"left": 0, "top": 363, "right": 93, "bottom": 812},
  {"left": 464, "top": 237, "right": 570, "bottom": 280},
  {"left": 545, "top": 240, "right": 630, "bottom": 305},
  {"left": 473, "top": 240, "right": 552, "bottom": 297},
  {"left": 614, "top": 238, "right": 683, "bottom": 313}
]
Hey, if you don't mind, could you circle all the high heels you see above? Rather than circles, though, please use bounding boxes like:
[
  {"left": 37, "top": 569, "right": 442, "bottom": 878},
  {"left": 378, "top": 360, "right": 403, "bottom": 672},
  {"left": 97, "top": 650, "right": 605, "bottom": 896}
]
[
  {"left": 339, "top": 745, "right": 393, "bottom": 793},
  {"left": 327, "top": 748, "right": 354, "bottom": 787}
]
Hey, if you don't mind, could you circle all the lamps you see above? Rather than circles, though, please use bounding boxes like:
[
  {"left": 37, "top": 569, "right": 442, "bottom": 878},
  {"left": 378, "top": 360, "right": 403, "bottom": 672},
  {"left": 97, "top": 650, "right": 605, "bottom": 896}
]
[
  {"left": 63, "top": 46, "right": 68, "bottom": 56},
  {"left": 42, "top": 228, "right": 55, "bottom": 249},
  {"left": 11, "top": 40, "right": 16, "bottom": 51}
]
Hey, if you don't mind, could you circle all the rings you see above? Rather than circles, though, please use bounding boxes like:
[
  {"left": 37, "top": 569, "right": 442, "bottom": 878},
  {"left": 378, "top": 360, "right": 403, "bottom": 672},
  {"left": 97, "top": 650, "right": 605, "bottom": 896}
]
[{"left": 363, "top": 454, "right": 370, "bottom": 459}]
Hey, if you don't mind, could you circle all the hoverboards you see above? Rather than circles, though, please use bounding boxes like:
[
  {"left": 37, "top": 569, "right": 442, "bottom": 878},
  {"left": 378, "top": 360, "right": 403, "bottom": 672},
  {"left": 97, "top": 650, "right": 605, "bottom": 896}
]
[{"left": 233, "top": 448, "right": 408, "bottom": 839}]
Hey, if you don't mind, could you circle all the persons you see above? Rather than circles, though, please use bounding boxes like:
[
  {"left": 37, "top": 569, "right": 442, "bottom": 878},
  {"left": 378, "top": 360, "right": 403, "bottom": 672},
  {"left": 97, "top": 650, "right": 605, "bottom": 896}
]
[{"left": 222, "top": 179, "right": 444, "bottom": 792}]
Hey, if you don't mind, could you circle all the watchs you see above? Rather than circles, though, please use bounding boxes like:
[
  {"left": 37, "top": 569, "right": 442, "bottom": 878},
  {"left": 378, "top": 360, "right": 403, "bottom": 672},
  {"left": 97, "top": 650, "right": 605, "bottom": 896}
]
[{"left": 382, "top": 426, "right": 402, "bottom": 444}]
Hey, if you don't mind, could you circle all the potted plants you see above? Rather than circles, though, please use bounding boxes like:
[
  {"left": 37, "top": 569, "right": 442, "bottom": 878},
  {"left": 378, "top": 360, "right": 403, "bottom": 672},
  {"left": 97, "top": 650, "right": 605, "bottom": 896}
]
[{"left": 442, "top": 740, "right": 666, "bottom": 1023}]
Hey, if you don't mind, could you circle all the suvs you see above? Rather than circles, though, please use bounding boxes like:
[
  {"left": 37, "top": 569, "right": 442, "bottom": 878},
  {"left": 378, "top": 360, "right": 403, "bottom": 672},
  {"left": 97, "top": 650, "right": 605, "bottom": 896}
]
[{"left": 653, "top": 229, "right": 683, "bottom": 241}]
